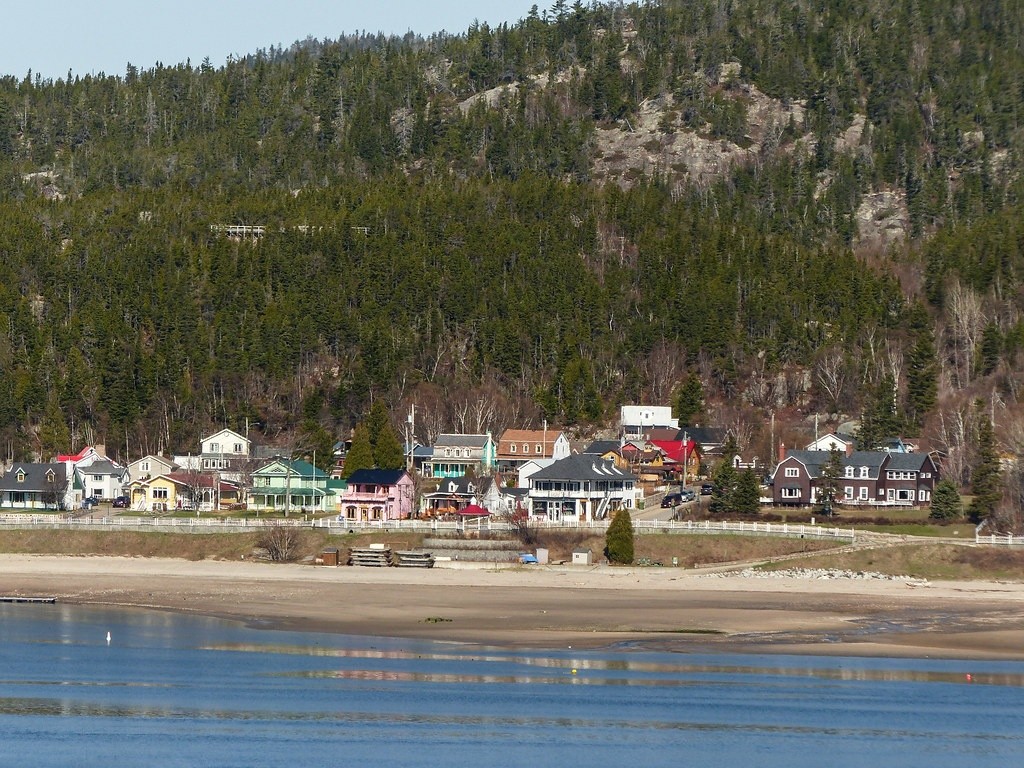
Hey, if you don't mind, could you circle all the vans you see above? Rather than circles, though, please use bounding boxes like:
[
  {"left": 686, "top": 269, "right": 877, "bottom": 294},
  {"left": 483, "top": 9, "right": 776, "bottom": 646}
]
[
  {"left": 660, "top": 493, "right": 681, "bottom": 508},
  {"left": 701, "top": 483, "right": 715, "bottom": 494}
]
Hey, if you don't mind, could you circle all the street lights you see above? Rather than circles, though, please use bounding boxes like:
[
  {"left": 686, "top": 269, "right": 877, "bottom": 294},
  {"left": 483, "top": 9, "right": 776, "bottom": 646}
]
[{"left": 681, "top": 430, "right": 688, "bottom": 491}]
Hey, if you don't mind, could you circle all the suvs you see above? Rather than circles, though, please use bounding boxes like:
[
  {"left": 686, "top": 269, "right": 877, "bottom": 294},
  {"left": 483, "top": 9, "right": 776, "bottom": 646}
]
[{"left": 112, "top": 496, "right": 130, "bottom": 508}]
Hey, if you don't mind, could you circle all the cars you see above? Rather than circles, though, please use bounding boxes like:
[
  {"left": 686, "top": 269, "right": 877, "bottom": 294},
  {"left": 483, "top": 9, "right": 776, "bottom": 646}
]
[
  {"left": 679, "top": 490, "right": 695, "bottom": 502},
  {"left": 82, "top": 497, "right": 98, "bottom": 508}
]
